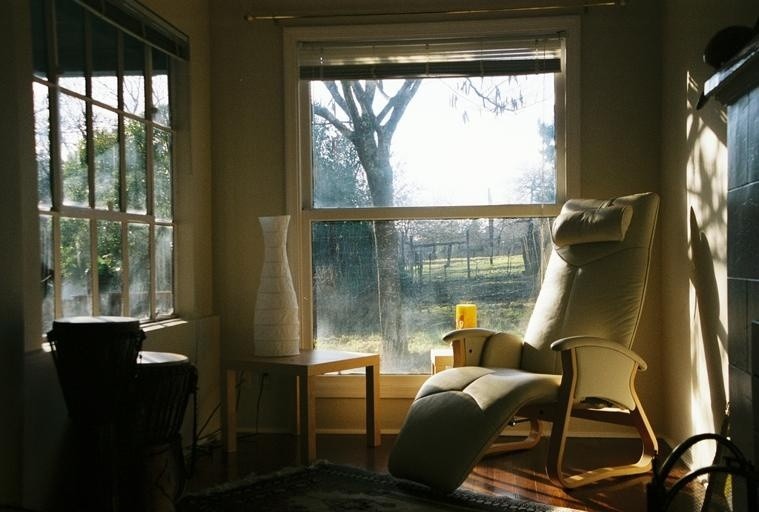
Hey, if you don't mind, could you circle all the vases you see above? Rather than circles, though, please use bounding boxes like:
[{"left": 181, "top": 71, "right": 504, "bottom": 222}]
[{"left": 252, "top": 215, "right": 301, "bottom": 358}]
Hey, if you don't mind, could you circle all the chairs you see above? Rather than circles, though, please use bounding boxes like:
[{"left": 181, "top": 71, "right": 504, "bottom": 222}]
[{"left": 387, "top": 192, "right": 660, "bottom": 493}]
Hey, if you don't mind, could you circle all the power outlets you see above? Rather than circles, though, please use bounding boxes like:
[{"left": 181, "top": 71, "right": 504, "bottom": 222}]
[{"left": 236, "top": 369, "right": 272, "bottom": 391}]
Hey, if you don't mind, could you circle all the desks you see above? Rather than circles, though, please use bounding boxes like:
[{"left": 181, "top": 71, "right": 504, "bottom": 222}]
[
  {"left": 220, "top": 353, "right": 381, "bottom": 483},
  {"left": 428, "top": 346, "right": 453, "bottom": 373}
]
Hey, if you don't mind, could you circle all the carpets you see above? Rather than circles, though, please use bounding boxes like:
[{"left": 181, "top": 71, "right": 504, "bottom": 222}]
[{"left": 175, "top": 459, "right": 586, "bottom": 512}]
